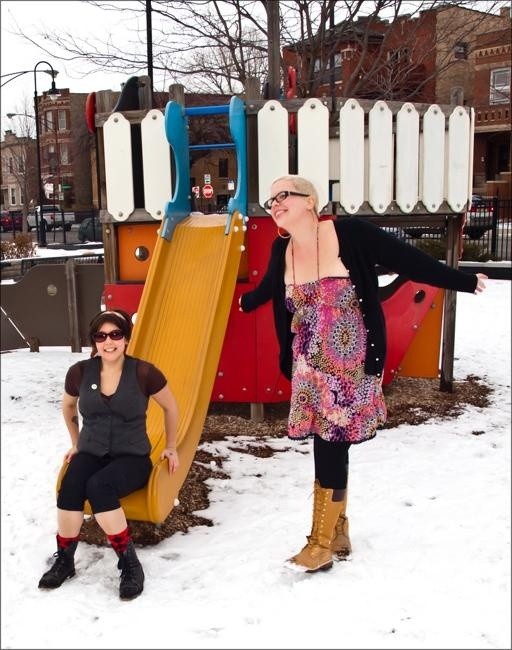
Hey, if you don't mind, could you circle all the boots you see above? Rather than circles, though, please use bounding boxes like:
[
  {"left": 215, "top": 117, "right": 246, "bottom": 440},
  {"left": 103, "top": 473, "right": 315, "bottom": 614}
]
[
  {"left": 111, "top": 537, "right": 144, "bottom": 601},
  {"left": 284, "top": 477, "right": 353, "bottom": 572},
  {"left": 39, "top": 538, "right": 78, "bottom": 588}
]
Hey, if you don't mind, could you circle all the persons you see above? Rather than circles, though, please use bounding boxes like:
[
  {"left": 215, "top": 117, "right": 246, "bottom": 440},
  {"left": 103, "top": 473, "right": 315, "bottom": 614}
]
[
  {"left": 239, "top": 176, "right": 489, "bottom": 574},
  {"left": 37, "top": 310, "right": 181, "bottom": 602}
]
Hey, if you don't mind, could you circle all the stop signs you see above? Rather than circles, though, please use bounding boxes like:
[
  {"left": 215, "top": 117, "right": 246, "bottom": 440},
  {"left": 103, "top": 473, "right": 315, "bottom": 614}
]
[{"left": 202, "top": 185, "right": 214, "bottom": 198}]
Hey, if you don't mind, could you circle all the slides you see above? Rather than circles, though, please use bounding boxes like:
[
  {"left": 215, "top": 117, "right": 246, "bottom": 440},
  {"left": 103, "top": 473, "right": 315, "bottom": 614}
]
[{"left": 57, "top": 211, "right": 249, "bottom": 523}]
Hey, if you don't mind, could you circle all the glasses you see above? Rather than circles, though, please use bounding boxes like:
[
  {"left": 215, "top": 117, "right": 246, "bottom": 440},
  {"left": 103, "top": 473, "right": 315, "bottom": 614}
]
[
  {"left": 264, "top": 189, "right": 310, "bottom": 211},
  {"left": 90, "top": 327, "right": 127, "bottom": 343}
]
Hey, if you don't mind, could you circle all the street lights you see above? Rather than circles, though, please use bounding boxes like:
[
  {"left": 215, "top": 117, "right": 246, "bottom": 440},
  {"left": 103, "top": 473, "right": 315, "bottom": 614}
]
[
  {"left": 7, "top": 112, "right": 60, "bottom": 184},
  {"left": 34, "top": 61, "right": 61, "bottom": 246}
]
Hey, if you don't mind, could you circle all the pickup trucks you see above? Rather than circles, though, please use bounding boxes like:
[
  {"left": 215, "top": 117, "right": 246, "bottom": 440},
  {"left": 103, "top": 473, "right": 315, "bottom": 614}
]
[{"left": 27, "top": 204, "right": 74, "bottom": 231}]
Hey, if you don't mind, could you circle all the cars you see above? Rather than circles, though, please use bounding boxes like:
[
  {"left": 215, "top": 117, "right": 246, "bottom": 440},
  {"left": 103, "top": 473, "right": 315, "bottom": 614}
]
[
  {"left": 78, "top": 217, "right": 102, "bottom": 244},
  {"left": 1, "top": 210, "right": 22, "bottom": 233},
  {"left": 404, "top": 195, "right": 493, "bottom": 239}
]
